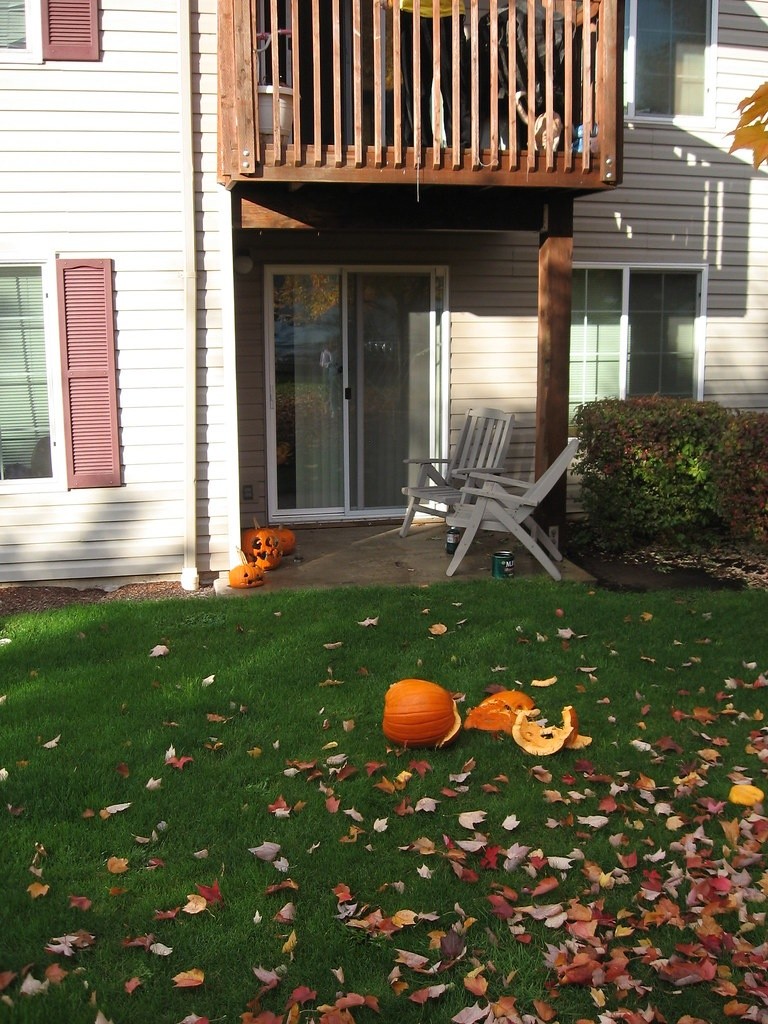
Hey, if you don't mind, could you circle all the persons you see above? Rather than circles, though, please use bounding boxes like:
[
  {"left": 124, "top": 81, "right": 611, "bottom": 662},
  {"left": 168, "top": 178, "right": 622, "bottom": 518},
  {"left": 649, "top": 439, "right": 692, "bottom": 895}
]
[{"left": 380, "top": 0, "right": 471, "bottom": 148}]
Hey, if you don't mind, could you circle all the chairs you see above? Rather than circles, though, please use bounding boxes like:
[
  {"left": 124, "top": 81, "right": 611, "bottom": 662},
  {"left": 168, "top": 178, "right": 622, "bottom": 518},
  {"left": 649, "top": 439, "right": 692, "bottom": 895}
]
[
  {"left": 445, "top": 438, "right": 581, "bottom": 580},
  {"left": 399, "top": 407, "right": 516, "bottom": 537}
]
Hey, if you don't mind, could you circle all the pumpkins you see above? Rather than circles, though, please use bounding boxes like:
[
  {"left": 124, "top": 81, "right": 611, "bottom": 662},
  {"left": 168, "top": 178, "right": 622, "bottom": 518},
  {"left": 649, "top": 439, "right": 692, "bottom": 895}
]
[{"left": 383, "top": 679, "right": 462, "bottom": 748}]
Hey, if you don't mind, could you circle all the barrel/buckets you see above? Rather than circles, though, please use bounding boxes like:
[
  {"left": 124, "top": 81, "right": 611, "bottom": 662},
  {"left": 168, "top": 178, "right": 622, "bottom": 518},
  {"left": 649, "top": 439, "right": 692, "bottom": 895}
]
[{"left": 491, "top": 552, "right": 515, "bottom": 579}]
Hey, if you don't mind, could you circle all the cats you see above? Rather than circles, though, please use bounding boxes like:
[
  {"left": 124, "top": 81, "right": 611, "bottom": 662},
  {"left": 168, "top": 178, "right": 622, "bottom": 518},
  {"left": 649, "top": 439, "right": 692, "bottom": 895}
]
[{"left": 515, "top": 91, "right": 563, "bottom": 152}]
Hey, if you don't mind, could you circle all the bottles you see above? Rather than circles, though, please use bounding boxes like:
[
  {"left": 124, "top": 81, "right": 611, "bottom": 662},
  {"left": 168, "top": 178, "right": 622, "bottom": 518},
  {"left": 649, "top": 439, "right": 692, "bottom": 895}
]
[{"left": 446, "top": 527, "right": 461, "bottom": 553}]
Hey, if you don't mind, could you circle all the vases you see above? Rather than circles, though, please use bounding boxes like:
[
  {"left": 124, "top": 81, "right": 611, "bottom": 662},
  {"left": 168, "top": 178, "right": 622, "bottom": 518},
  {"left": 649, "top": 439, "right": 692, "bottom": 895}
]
[{"left": 258, "top": 86, "right": 300, "bottom": 144}]
